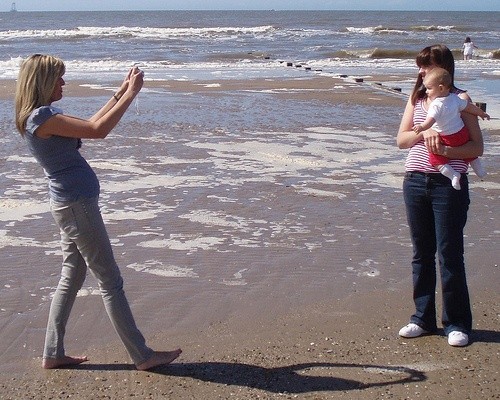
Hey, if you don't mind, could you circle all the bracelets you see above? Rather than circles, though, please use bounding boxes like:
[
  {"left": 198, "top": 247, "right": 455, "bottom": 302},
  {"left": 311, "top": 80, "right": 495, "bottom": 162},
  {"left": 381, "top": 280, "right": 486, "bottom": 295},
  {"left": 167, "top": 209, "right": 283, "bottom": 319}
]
[
  {"left": 113, "top": 94, "right": 120, "bottom": 102},
  {"left": 444, "top": 145, "right": 447, "bottom": 156}
]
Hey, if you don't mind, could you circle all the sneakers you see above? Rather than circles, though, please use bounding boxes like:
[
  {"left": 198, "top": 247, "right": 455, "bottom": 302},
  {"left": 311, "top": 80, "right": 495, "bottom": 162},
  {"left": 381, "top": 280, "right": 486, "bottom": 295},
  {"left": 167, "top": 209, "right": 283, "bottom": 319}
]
[
  {"left": 399, "top": 323, "right": 423, "bottom": 337},
  {"left": 448, "top": 331, "right": 468, "bottom": 346}
]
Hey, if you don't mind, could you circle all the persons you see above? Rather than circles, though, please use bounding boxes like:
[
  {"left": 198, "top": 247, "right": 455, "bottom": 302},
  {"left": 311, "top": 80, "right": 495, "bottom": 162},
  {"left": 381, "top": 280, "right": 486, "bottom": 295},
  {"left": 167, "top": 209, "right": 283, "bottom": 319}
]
[
  {"left": 16, "top": 53, "right": 183, "bottom": 372},
  {"left": 396, "top": 43, "right": 484, "bottom": 347},
  {"left": 462, "top": 36, "right": 479, "bottom": 60},
  {"left": 411, "top": 66, "right": 491, "bottom": 190}
]
